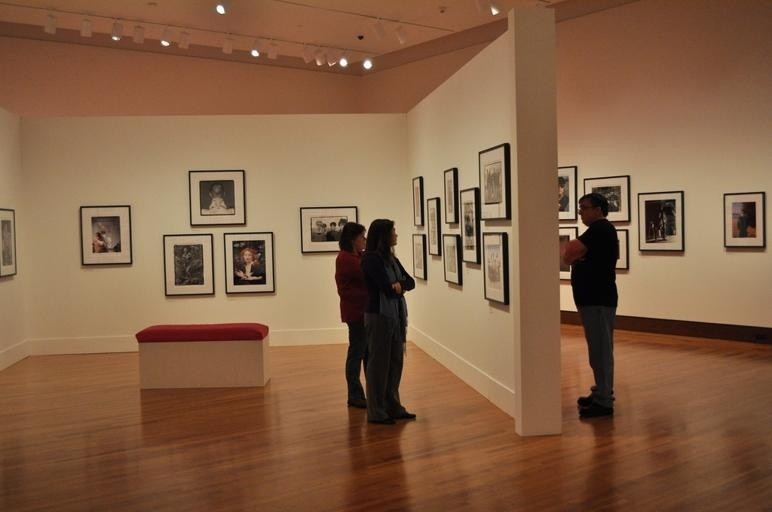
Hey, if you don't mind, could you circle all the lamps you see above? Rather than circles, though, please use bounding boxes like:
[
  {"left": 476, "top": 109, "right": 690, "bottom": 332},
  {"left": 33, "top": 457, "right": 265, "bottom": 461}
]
[
  {"left": 372, "top": 23, "right": 388, "bottom": 40},
  {"left": 268, "top": 42, "right": 279, "bottom": 60},
  {"left": 112, "top": 23, "right": 123, "bottom": 41},
  {"left": 300, "top": 45, "right": 314, "bottom": 64},
  {"left": 326, "top": 50, "right": 336, "bottom": 67},
  {"left": 363, "top": 57, "right": 375, "bottom": 70},
  {"left": 177, "top": 31, "right": 192, "bottom": 48},
  {"left": 314, "top": 50, "right": 325, "bottom": 66},
  {"left": 490, "top": 2, "right": 503, "bottom": 16},
  {"left": 134, "top": 26, "right": 144, "bottom": 44},
  {"left": 251, "top": 40, "right": 262, "bottom": 57},
  {"left": 340, "top": 56, "right": 349, "bottom": 68},
  {"left": 216, "top": 0, "right": 232, "bottom": 15},
  {"left": 44, "top": 15, "right": 57, "bottom": 34},
  {"left": 222, "top": 38, "right": 234, "bottom": 54},
  {"left": 160, "top": 26, "right": 175, "bottom": 47},
  {"left": 80, "top": 19, "right": 92, "bottom": 37},
  {"left": 395, "top": 29, "right": 408, "bottom": 44}
]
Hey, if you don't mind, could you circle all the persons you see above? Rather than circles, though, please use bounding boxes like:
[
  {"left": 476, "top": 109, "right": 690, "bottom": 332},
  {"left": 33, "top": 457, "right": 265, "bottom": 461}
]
[
  {"left": 361, "top": 219, "right": 416, "bottom": 425},
  {"left": 312, "top": 217, "right": 346, "bottom": 242},
  {"left": 236, "top": 248, "right": 264, "bottom": 281},
  {"left": 335, "top": 222, "right": 369, "bottom": 409},
  {"left": 208, "top": 183, "right": 228, "bottom": 209},
  {"left": 562, "top": 193, "right": 620, "bottom": 418},
  {"left": 737, "top": 209, "right": 750, "bottom": 237},
  {"left": 558, "top": 177, "right": 569, "bottom": 211}
]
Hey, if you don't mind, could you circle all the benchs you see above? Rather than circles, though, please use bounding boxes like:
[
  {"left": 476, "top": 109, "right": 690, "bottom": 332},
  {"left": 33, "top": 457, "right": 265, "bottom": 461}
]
[{"left": 135, "top": 323, "right": 270, "bottom": 388}]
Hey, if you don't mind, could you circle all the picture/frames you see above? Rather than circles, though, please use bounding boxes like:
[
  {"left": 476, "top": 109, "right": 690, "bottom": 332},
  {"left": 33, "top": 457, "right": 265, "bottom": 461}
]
[
  {"left": 300, "top": 206, "right": 358, "bottom": 254},
  {"left": 557, "top": 166, "right": 578, "bottom": 221},
  {"left": 723, "top": 191, "right": 766, "bottom": 248},
  {"left": 482, "top": 232, "right": 509, "bottom": 305},
  {"left": 613, "top": 229, "right": 629, "bottom": 270},
  {"left": 442, "top": 234, "right": 462, "bottom": 285},
  {"left": 412, "top": 234, "right": 427, "bottom": 280},
  {"left": 427, "top": 197, "right": 441, "bottom": 256},
  {"left": 412, "top": 176, "right": 424, "bottom": 226},
  {"left": 638, "top": 191, "right": 684, "bottom": 252},
  {"left": 559, "top": 227, "right": 578, "bottom": 281},
  {"left": 444, "top": 168, "right": 458, "bottom": 224},
  {"left": 584, "top": 175, "right": 631, "bottom": 223},
  {"left": 224, "top": 232, "right": 275, "bottom": 294},
  {"left": 460, "top": 187, "right": 480, "bottom": 264},
  {"left": 479, "top": 142, "right": 511, "bottom": 220},
  {"left": 80, "top": 206, "right": 133, "bottom": 266},
  {"left": 188, "top": 170, "right": 247, "bottom": 226},
  {"left": 0, "top": 208, "right": 17, "bottom": 278},
  {"left": 163, "top": 234, "right": 215, "bottom": 296}
]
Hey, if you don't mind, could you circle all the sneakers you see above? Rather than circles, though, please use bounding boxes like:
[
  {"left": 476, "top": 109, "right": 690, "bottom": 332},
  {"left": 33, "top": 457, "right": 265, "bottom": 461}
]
[
  {"left": 367, "top": 414, "right": 395, "bottom": 425},
  {"left": 347, "top": 397, "right": 367, "bottom": 409},
  {"left": 576, "top": 394, "right": 595, "bottom": 407},
  {"left": 390, "top": 409, "right": 417, "bottom": 420},
  {"left": 579, "top": 403, "right": 614, "bottom": 417}
]
